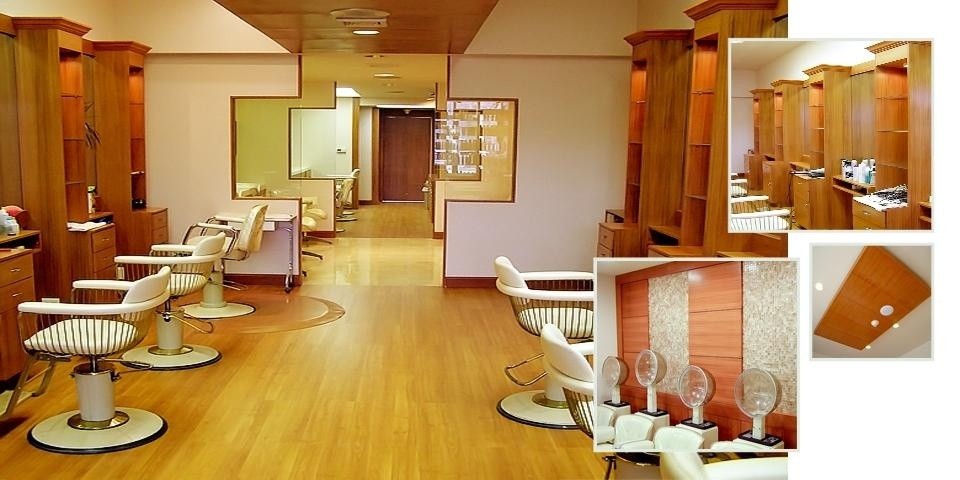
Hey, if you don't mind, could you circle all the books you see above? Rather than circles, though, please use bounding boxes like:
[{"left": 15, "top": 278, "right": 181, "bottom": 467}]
[{"left": 66, "top": 221, "right": 108, "bottom": 234}]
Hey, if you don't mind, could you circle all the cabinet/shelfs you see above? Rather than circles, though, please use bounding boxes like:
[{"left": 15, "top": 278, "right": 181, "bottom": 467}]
[{"left": 744, "top": 42, "right": 931, "bottom": 229}]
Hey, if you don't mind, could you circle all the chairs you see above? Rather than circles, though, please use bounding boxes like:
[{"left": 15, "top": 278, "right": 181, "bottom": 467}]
[
  {"left": 731, "top": 173, "right": 793, "bottom": 230},
  {"left": 301, "top": 169, "right": 359, "bottom": 277},
  {"left": 596, "top": 406, "right": 758, "bottom": 450},
  {"left": 494, "top": 256, "right": 592, "bottom": 441}
]
